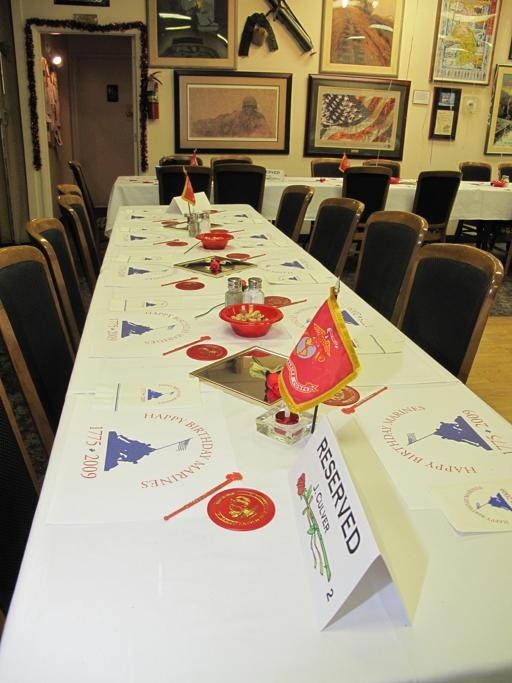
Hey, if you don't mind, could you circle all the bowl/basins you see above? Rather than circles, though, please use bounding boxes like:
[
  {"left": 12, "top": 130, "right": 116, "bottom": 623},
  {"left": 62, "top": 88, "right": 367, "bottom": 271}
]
[
  {"left": 389, "top": 177, "right": 400, "bottom": 184},
  {"left": 492, "top": 180, "right": 505, "bottom": 186},
  {"left": 198, "top": 232, "right": 234, "bottom": 249},
  {"left": 221, "top": 302, "right": 284, "bottom": 336}
]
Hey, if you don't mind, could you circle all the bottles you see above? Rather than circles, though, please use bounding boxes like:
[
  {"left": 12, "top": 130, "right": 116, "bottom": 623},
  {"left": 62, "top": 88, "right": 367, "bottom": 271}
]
[
  {"left": 501, "top": 175, "right": 509, "bottom": 185},
  {"left": 186, "top": 211, "right": 211, "bottom": 237},
  {"left": 226, "top": 276, "right": 265, "bottom": 305}
]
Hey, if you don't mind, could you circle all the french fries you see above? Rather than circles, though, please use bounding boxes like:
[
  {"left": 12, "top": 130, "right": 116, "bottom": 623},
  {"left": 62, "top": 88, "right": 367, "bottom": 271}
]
[
  {"left": 229, "top": 304, "right": 269, "bottom": 323},
  {"left": 204, "top": 236, "right": 225, "bottom": 239}
]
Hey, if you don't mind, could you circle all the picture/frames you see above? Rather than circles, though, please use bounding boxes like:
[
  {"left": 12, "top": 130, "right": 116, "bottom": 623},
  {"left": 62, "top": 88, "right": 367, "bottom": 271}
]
[
  {"left": 315, "top": 0, "right": 408, "bottom": 78},
  {"left": 144, "top": 0, "right": 239, "bottom": 69},
  {"left": 302, "top": 73, "right": 411, "bottom": 160},
  {"left": 427, "top": 86, "right": 463, "bottom": 141},
  {"left": 483, "top": 63, "right": 512, "bottom": 156},
  {"left": 53, "top": 0, "right": 109, "bottom": 7},
  {"left": 171, "top": 66, "right": 294, "bottom": 156}
]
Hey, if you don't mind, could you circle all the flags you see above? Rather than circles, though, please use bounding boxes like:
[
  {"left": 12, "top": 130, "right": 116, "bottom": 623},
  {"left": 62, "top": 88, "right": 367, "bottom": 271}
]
[
  {"left": 318, "top": 96, "right": 396, "bottom": 143},
  {"left": 180, "top": 169, "right": 196, "bottom": 204},
  {"left": 271, "top": 288, "right": 362, "bottom": 416}
]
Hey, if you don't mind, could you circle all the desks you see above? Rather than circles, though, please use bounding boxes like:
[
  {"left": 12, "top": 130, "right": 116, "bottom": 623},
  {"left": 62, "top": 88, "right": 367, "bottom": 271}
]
[
  {"left": 104, "top": 175, "right": 511, "bottom": 238},
  {"left": 0, "top": 203, "right": 512, "bottom": 682}
]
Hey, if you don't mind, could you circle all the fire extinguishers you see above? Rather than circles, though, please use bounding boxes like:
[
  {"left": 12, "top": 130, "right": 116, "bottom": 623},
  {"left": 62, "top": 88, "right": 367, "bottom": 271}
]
[{"left": 146, "top": 71, "right": 163, "bottom": 120}]
